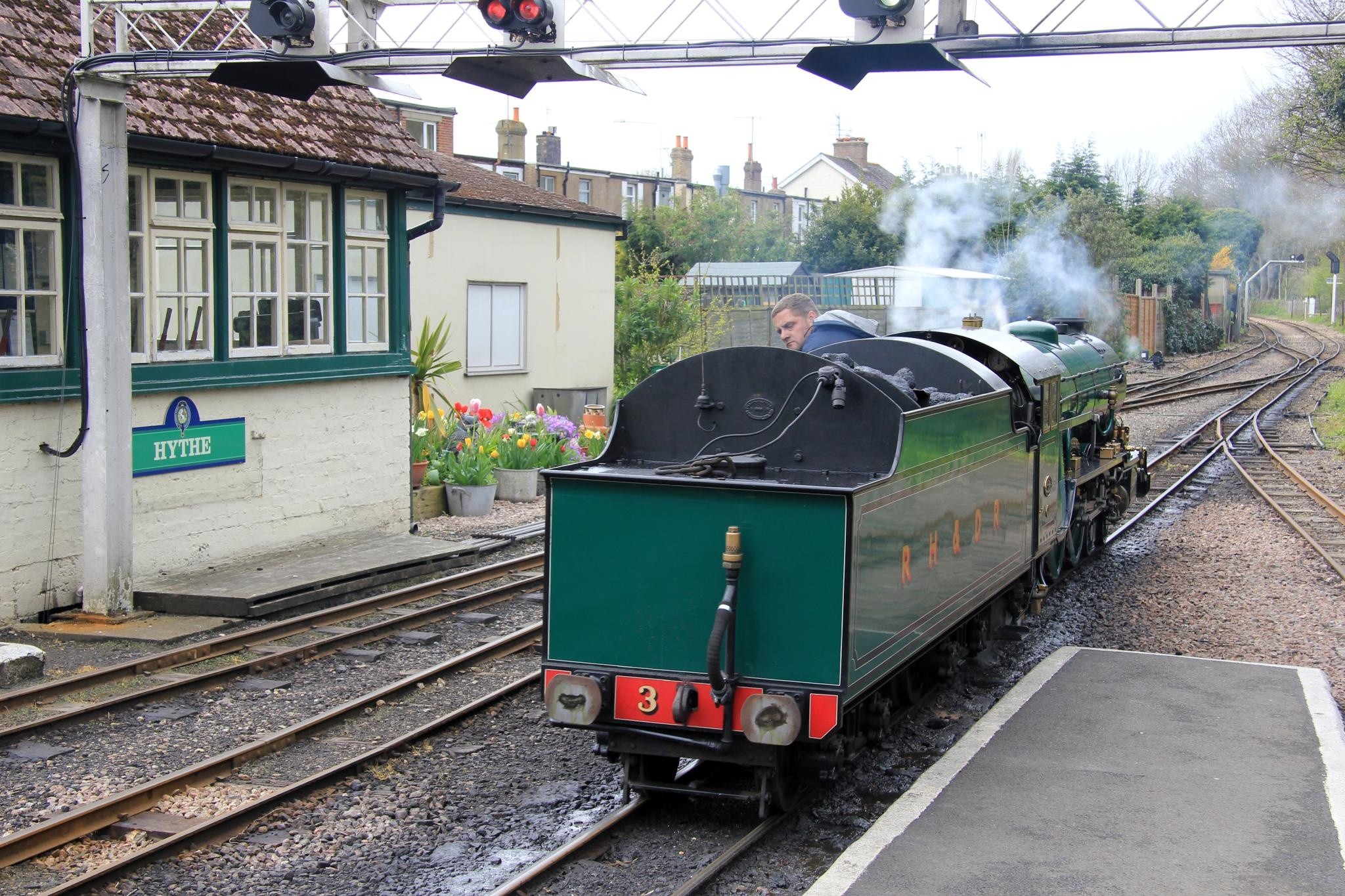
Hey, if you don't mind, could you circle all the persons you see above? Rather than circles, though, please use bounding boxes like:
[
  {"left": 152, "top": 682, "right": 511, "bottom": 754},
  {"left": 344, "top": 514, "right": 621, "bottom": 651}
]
[{"left": 769, "top": 292, "right": 881, "bottom": 355}]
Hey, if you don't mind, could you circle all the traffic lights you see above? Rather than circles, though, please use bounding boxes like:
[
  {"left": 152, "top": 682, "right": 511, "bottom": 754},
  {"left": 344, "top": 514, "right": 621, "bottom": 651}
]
[
  {"left": 248, "top": 0, "right": 314, "bottom": 38},
  {"left": 838, "top": 0, "right": 914, "bottom": 21},
  {"left": 478, "top": 0, "right": 554, "bottom": 30}
]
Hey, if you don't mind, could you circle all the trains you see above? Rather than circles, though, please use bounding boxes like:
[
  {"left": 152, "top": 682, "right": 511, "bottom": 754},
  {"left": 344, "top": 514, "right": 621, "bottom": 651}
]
[{"left": 542, "top": 317, "right": 1154, "bottom": 820}]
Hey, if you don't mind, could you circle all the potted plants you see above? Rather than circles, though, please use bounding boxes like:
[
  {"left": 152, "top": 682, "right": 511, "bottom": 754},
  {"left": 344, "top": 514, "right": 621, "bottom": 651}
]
[{"left": 411, "top": 399, "right": 601, "bottom": 517}]
